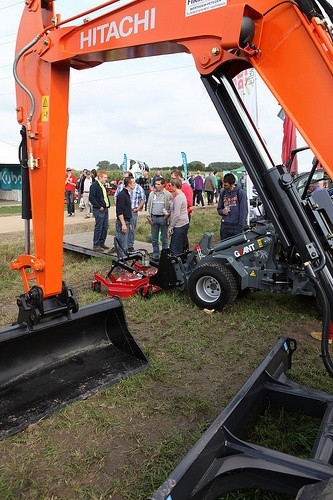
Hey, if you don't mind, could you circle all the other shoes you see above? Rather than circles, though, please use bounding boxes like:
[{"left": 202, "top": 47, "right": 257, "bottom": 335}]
[
  {"left": 94, "top": 247, "right": 104, "bottom": 252},
  {"left": 108, "top": 247, "right": 117, "bottom": 253},
  {"left": 128, "top": 247, "right": 135, "bottom": 251},
  {"left": 308, "top": 331, "right": 333, "bottom": 345},
  {"left": 101, "top": 244, "right": 109, "bottom": 250}
]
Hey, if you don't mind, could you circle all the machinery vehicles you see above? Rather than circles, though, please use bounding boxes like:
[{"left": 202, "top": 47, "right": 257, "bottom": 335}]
[
  {"left": 91, "top": 146, "right": 333, "bottom": 311},
  {"left": 0, "top": 0, "right": 333, "bottom": 500}
]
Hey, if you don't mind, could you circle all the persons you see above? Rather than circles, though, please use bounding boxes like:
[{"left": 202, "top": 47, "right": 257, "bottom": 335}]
[
  {"left": 217, "top": 173, "right": 249, "bottom": 240},
  {"left": 240, "top": 173, "right": 247, "bottom": 190},
  {"left": 189, "top": 175, "right": 197, "bottom": 206},
  {"left": 195, "top": 171, "right": 205, "bottom": 208},
  {"left": 146, "top": 177, "right": 174, "bottom": 261},
  {"left": 79, "top": 170, "right": 95, "bottom": 218},
  {"left": 108, "top": 171, "right": 147, "bottom": 252},
  {"left": 139, "top": 170, "right": 151, "bottom": 211},
  {"left": 170, "top": 170, "right": 194, "bottom": 252},
  {"left": 91, "top": 169, "right": 97, "bottom": 184},
  {"left": 116, "top": 176, "right": 136, "bottom": 261},
  {"left": 89, "top": 170, "right": 111, "bottom": 252},
  {"left": 213, "top": 171, "right": 220, "bottom": 203},
  {"left": 232, "top": 172, "right": 239, "bottom": 186},
  {"left": 218, "top": 176, "right": 223, "bottom": 195},
  {"left": 168, "top": 178, "right": 190, "bottom": 256},
  {"left": 76, "top": 174, "right": 85, "bottom": 212},
  {"left": 204, "top": 173, "right": 217, "bottom": 205},
  {"left": 151, "top": 171, "right": 163, "bottom": 188},
  {"left": 65, "top": 168, "right": 78, "bottom": 216}
]
